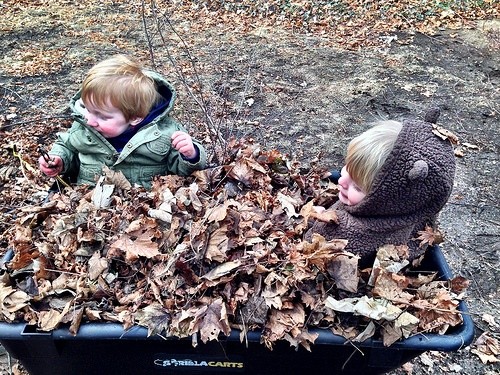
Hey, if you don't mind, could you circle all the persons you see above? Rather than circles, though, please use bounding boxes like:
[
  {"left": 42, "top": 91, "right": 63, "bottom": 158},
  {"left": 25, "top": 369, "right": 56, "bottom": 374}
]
[
  {"left": 36, "top": 53, "right": 204, "bottom": 191},
  {"left": 302, "top": 108, "right": 454, "bottom": 277}
]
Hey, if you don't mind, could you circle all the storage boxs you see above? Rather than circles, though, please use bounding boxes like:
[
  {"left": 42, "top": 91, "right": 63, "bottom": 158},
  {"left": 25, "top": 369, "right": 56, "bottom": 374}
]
[{"left": 1, "top": 170, "right": 474, "bottom": 375}]
